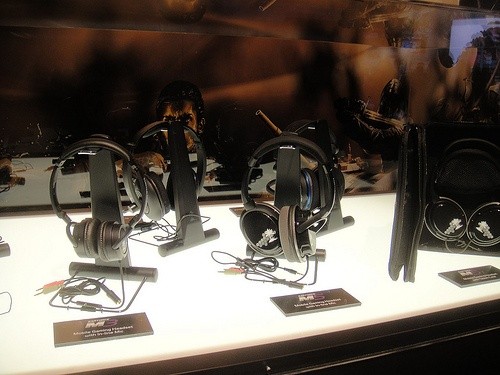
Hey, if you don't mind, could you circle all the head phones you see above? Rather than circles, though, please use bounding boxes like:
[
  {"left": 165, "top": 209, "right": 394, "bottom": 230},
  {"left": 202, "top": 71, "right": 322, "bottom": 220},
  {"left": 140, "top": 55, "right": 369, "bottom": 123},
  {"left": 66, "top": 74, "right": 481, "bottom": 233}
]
[
  {"left": 241, "top": 135, "right": 337, "bottom": 260},
  {"left": 424, "top": 136, "right": 500, "bottom": 251},
  {"left": 125, "top": 118, "right": 208, "bottom": 220},
  {"left": 53, "top": 138, "right": 147, "bottom": 259}
]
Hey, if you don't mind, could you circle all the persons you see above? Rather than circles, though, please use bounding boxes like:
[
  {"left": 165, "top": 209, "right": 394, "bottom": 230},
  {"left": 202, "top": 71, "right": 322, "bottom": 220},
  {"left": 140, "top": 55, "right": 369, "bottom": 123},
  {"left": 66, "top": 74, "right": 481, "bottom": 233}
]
[{"left": 154, "top": 80, "right": 227, "bottom": 209}]
